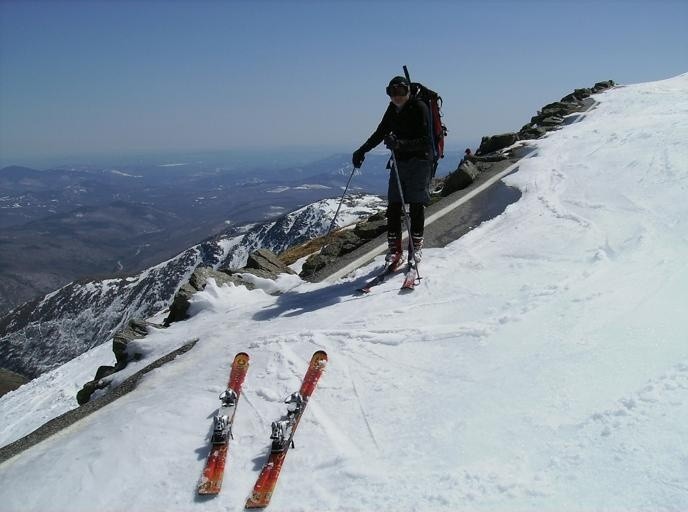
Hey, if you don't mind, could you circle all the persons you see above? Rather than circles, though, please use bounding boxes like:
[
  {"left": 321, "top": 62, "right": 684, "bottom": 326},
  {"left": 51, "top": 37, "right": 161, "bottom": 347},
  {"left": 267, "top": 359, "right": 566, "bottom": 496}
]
[{"left": 352, "top": 76, "right": 434, "bottom": 272}]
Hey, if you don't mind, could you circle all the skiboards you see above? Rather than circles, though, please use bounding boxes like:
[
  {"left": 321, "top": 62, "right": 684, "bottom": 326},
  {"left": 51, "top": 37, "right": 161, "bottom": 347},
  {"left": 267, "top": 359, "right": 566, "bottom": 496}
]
[
  {"left": 199, "top": 352, "right": 327, "bottom": 508},
  {"left": 357, "top": 254, "right": 421, "bottom": 294}
]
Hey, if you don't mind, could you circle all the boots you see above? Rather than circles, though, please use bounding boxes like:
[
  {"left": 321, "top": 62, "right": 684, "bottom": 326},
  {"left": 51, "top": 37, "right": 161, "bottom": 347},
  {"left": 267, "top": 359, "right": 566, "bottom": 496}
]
[
  {"left": 385, "top": 232, "right": 402, "bottom": 261},
  {"left": 409, "top": 234, "right": 423, "bottom": 262}
]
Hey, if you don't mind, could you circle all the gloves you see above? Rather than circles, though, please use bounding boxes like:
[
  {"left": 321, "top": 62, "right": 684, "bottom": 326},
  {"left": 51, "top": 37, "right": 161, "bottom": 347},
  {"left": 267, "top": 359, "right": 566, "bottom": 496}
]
[
  {"left": 385, "top": 134, "right": 402, "bottom": 150},
  {"left": 353, "top": 148, "right": 365, "bottom": 168}
]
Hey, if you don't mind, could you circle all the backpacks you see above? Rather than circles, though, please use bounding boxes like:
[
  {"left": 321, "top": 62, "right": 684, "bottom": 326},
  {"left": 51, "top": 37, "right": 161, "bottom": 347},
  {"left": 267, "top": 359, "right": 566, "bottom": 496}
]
[{"left": 409, "top": 83, "right": 444, "bottom": 176}]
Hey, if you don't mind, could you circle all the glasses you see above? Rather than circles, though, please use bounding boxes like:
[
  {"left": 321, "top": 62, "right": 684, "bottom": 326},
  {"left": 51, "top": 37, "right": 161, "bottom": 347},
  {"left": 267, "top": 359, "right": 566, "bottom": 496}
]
[{"left": 386, "top": 86, "right": 408, "bottom": 96}]
